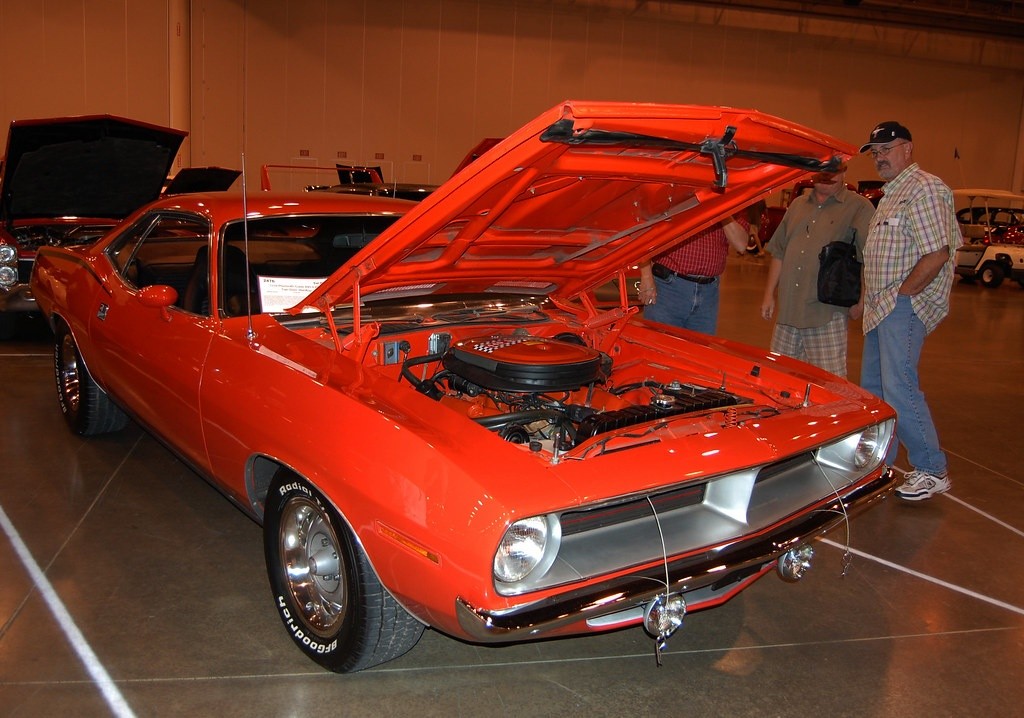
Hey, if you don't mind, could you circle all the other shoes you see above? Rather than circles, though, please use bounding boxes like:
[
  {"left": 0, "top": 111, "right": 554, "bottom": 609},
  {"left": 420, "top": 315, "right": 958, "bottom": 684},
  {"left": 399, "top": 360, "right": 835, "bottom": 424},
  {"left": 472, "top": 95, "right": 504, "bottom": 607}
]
[
  {"left": 754, "top": 252, "right": 766, "bottom": 257},
  {"left": 737, "top": 251, "right": 745, "bottom": 256}
]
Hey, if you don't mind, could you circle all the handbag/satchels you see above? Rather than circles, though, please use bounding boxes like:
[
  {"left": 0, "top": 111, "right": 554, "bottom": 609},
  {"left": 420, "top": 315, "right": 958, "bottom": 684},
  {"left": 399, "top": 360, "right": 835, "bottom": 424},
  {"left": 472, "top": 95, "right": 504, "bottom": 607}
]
[{"left": 817, "top": 229, "right": 862, "bottom": 307}]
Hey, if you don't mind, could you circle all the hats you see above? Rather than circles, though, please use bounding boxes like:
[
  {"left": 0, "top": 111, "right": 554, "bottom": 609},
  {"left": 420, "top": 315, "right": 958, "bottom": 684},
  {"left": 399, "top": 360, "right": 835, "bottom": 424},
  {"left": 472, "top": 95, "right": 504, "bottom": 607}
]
[{"left": 859, "top": 121, "right": 911, "bottom": 152}]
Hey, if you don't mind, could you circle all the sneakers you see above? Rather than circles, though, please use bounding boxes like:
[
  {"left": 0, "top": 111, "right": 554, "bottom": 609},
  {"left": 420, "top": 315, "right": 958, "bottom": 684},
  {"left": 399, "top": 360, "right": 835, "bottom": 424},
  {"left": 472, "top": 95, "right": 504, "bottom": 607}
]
[{"left": 894, "top": 468, "right": 951, "bottom": 501}]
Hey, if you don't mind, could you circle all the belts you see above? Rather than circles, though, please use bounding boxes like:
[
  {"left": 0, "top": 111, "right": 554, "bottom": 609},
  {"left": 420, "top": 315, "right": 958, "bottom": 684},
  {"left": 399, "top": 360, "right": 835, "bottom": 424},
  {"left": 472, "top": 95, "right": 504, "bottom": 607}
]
[{"left": 670, "top": 271, "right": 715, "bottom": 285}]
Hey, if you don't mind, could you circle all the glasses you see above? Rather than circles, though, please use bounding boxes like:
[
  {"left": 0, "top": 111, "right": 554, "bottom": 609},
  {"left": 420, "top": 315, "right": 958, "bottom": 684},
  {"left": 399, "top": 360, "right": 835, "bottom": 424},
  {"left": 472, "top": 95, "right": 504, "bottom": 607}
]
[{"left": 866, "top": 141, "right": 909, "bottom": 159}]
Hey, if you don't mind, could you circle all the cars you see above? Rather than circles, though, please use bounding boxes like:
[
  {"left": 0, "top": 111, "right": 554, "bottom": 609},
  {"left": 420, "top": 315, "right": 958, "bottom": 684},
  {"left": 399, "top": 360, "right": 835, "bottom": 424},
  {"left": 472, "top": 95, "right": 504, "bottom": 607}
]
[
  {"left": 33, "top": 96, "right": 901, "bottom": 677},
  {"left": 957, "top": 205, "right": 1024, "bottom": 247},
  {"left": 0, "top": 113, "right": 189, "bottom": 343},
  {"left": 156, "top": 166, "right": 244, "bottom": 197}
]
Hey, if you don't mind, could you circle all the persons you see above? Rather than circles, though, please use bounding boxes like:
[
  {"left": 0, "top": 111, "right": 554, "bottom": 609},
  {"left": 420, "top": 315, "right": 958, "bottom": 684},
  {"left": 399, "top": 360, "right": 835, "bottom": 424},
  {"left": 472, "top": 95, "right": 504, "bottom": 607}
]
[
  {"left": 638, "top": 207, "right": 750, "bottom": 336},
  {"left": 859, "top": 121, "right": 966, "bottom": 500},
  {"left": 760, "top": 159, "right": 876, "bottom": 382}
]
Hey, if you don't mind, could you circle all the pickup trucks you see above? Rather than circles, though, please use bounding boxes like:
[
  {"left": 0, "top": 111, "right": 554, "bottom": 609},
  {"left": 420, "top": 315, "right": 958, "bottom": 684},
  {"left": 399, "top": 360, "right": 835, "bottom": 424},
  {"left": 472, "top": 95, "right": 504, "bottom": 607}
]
[{"left": 743, "top": 182, "right": 883, "bottom": 253}]
